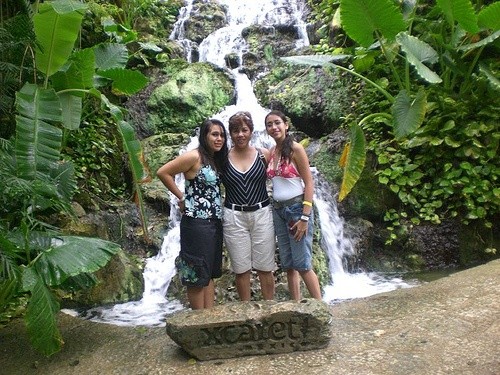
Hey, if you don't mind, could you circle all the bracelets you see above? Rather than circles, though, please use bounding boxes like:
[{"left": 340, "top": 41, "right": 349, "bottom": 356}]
[
  {"left": 178, "top": 194, "right": 185, "bottom": 202},
  {"left": 302, "top": 201, "right": 313, "bottom": 208},
  {"left": 300, "top": 214, "right": 309, "bottom": 222}
]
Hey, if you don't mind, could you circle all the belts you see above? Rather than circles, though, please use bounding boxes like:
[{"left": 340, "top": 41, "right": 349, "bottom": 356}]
[
  {"left": 273, "top": 194, "right": 304, "bottom": 208},
  {"left": 223, "top": 198, "right": 272, "bottom": 213}
]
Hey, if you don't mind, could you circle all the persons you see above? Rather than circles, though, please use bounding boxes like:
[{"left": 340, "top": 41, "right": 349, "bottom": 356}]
[
  {"left": 156, "top": 118, "right": 229, "bottom": 309},
  {"left": 267, "top": 110, "right": 321, "bottom": 301},
  {"left": 222, "top": 112, "right": 275, "bottom": 302}
]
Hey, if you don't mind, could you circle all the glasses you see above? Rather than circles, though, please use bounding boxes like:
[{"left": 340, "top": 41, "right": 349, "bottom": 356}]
[{"left": 235, "top": 113, "right": 251, "bottom": 121}]
